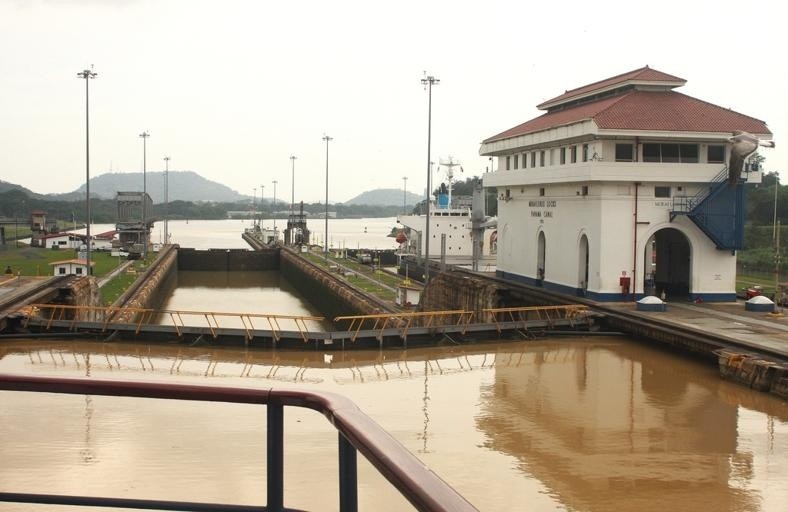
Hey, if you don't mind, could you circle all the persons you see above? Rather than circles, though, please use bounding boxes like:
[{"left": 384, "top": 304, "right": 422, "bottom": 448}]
[
  {"left": 5, "top": 266, "right": 12, "bottom": 274},
  {"left": 620, "top": 269, "right": 630, "bottom": 296}
]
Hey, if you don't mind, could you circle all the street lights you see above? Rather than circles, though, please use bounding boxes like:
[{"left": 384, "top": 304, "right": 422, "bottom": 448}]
[
  {"left": 78, "top": 70, "right": 97, "bottom": 275},
  {"left": 162, "top": 158, "right": 170, "bottom": 244},
  {"left": 290, "top": 156, "right": 297, "bottom": 244},
  {"left": 323, "top": 136, "right": 333, "bottom": 259},
  {"left": 140, "top": 132, "right": 150, "bottom": 257},
  {"left": 403, "top": 77, "right": 440, "bottom": 284},
  {"left": 253, "top": 181, "right": 277, "bottom": 232}
]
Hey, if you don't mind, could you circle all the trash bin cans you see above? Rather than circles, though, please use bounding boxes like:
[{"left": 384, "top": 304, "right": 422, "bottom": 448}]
[
  {"left": 301, "top": 246, "right": 307, "bottom": 252},
  {"left": 153, "top": 242, "right": 159, "bottom": 253}
]
